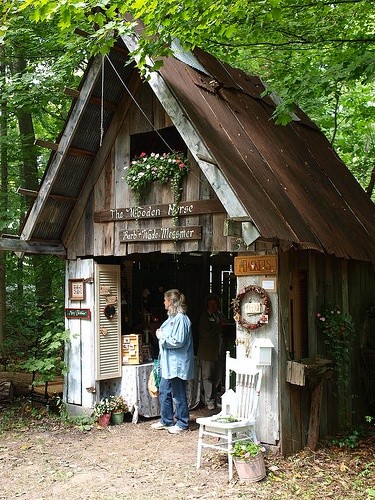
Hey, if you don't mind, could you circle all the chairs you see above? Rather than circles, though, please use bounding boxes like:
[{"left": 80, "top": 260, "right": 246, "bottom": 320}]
[{"left": 196, "top": 351, "right": 263, "bottom": 483}]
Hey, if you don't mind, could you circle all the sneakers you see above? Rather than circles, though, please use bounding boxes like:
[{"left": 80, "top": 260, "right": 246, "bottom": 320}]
[
  {"left": 168, "top": 424, "right": 184, "bottom": 433},
  {"left": 151, "top": 422, "right": 173, "bottom": 429}
]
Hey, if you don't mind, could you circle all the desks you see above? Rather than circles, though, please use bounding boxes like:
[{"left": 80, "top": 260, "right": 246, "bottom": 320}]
[{"left": 101, "top": 356, "right": 201, "bottom": 425}]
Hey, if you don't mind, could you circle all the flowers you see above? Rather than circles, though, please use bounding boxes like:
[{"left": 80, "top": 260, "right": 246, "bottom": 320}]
[
  {"left": 90, "top": 394, "right": 129, "bottom": 418},
  {"left": 121, "top": 152, "right": 193, "bottom": 243},
  {"left": 230, "top": 285, "right": 271, "bottom": 329},
  {"left": 229, "top": 439, "right": 266, "bottom": 468}
]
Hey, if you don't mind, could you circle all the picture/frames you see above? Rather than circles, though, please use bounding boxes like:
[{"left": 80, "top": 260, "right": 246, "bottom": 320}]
[
  {"left": 65, "top": 308, "right": 90, "bottom": 319},
  {"left": 69, "top": 278, "right": 86, "bottom": 301}
]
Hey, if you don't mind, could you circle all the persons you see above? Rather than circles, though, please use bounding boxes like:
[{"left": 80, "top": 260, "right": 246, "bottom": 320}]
[
  {"left": 196, "top": 295, "right": 227, "bottom": 410},
  {"left": 150, "top": 289, "right": 195, "bottom": 434}
]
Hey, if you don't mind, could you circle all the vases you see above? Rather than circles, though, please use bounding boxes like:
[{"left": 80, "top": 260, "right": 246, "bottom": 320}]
[
  {"left": 98, "top": 414, "right": 111, "bottom": 426},
  {"left": 112, "top": 410, "right": 125, "bottom": 424}
]
